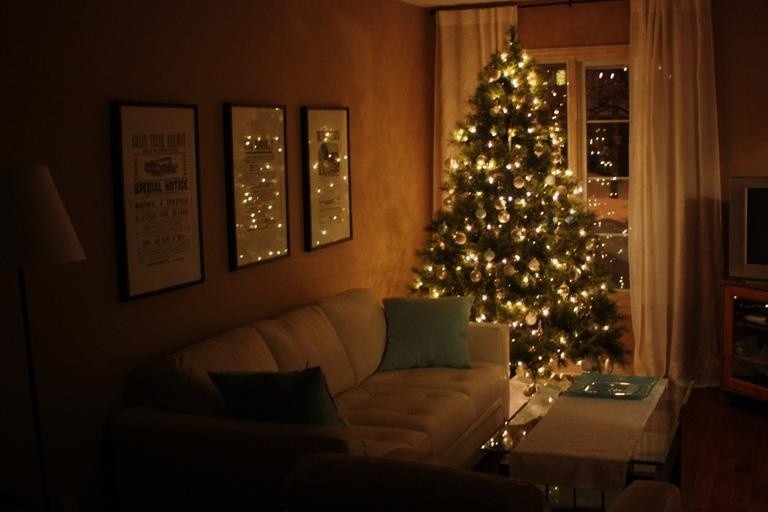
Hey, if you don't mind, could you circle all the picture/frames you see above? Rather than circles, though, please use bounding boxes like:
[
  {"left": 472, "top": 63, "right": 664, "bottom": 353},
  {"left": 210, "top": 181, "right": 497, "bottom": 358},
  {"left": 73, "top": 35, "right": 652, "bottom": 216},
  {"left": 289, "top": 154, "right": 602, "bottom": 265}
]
[
  {"left": 301, "top": 104, "right": 353, "bottom": 252},
  {"left": 110, "top": 100, "right": 205, "bottom": 298},
  {"left": 223, "top": 103, "right": 291, "bottom": 271}
]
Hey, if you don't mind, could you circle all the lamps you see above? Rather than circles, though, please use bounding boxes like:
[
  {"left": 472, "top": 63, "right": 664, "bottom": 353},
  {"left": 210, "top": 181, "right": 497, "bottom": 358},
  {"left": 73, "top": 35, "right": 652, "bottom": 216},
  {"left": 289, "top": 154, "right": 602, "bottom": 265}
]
[{"left": 0, "top": 168, "right": 80, "bottom": 512}]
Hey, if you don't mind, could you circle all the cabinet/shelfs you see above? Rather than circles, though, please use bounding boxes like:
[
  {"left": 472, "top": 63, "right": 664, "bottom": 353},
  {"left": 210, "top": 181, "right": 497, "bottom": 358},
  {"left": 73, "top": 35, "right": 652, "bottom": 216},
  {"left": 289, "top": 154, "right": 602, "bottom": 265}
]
[{"left": 722, "top": 282, "right": 768, "bottom": 405}]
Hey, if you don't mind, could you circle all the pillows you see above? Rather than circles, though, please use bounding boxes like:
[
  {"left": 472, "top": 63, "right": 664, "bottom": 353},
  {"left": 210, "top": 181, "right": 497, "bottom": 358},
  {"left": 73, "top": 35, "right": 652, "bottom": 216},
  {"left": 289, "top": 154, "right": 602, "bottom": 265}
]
[
  {"left": 208, "top": 366, "right": 352, "bottom": 427},
  {"left": 380, "top": 293, "right": 476, "bottom": 370}
]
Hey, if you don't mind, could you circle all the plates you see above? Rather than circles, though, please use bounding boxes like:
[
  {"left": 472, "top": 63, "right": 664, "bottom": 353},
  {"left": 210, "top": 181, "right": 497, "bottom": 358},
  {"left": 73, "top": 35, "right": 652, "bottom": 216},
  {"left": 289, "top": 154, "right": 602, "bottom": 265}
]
[{"left": 567, "top": 373, "right": 661, "bottom": 399}]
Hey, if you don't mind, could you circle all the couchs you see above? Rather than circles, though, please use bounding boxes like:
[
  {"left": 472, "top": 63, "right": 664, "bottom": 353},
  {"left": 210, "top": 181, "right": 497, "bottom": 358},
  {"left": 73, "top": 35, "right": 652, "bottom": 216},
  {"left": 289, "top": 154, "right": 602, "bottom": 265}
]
[
  {"left": 147, "top": 288, "right": 510, "bottom": 472},
  {"left": 112, "top": 415, "right": 683, "bottom": 512}
]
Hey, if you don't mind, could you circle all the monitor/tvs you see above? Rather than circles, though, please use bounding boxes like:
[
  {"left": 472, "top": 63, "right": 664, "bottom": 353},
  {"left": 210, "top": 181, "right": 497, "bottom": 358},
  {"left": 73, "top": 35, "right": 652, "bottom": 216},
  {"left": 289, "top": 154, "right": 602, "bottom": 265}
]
[{"left": 729, "top": 176, "right": 764, "bottom": 277}]
